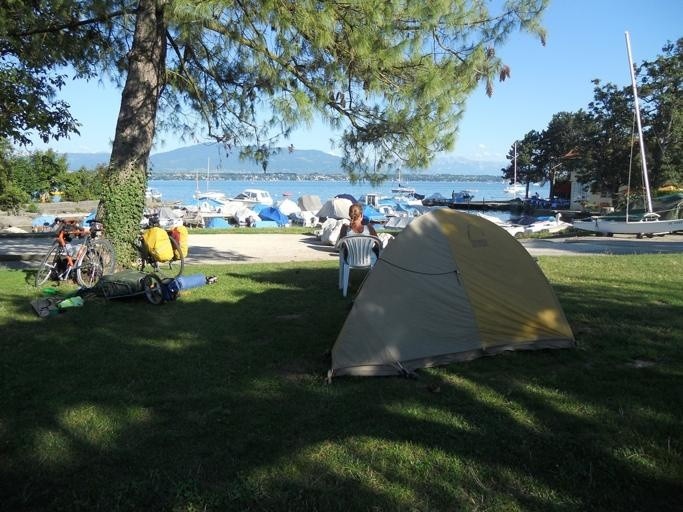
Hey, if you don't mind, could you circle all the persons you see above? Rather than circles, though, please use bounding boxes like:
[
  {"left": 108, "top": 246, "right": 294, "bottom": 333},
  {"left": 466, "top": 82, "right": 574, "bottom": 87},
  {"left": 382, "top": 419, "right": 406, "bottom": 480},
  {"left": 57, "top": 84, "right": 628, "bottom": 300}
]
[
  {"left": 452, "top": 190, "right": 455, "bottom": 199},
  {"left": 535, "top": 191, "right": 539, "bottom": 199},
  {"left": 338, "top": 203, "right": 379, "bottom": 265}
]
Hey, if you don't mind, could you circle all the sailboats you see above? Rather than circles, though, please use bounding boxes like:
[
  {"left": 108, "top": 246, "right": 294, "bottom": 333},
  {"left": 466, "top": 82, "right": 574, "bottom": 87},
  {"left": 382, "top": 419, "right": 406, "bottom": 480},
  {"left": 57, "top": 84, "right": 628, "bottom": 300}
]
[{"left": 571, "top": 30, "right": 683, "bottom": 238}]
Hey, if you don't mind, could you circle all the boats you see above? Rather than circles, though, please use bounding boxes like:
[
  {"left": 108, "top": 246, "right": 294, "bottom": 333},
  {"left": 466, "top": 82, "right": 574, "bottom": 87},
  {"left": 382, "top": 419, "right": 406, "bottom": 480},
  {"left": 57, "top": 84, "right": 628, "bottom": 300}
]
[
  {"left": 475, "top": 211, "right": 571, "bottom": 239},
  {"left": 503, "top": 182, "right": 525, "bottom": 193}
]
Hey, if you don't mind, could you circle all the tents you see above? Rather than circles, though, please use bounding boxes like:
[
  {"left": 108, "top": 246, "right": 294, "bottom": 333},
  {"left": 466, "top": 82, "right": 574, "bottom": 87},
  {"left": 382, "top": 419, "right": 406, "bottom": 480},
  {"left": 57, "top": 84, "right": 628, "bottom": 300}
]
[{"left": 327, "top": 207, "right": 576, "bottom": 379}]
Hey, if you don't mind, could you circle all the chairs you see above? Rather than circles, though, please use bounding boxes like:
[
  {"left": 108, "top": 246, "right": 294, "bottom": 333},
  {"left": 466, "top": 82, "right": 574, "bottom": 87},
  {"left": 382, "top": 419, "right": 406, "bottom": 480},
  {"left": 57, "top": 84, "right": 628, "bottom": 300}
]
[{"left": 335, "top": 234, "right": 383, "bottom": 298}]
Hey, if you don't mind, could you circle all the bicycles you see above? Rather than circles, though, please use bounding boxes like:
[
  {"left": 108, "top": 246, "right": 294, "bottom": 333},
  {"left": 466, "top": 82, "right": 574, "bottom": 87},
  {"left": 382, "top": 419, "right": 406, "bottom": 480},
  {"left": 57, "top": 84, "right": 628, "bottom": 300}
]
[
  {"left": 138, "top": 212, "right": 184, "bottom": 280},
  {"left": 96, "top": 275, "right": 166, "bottom": 305},
  {"left": 34, "top": 217, "right": 118, "bottom": 291}
]
